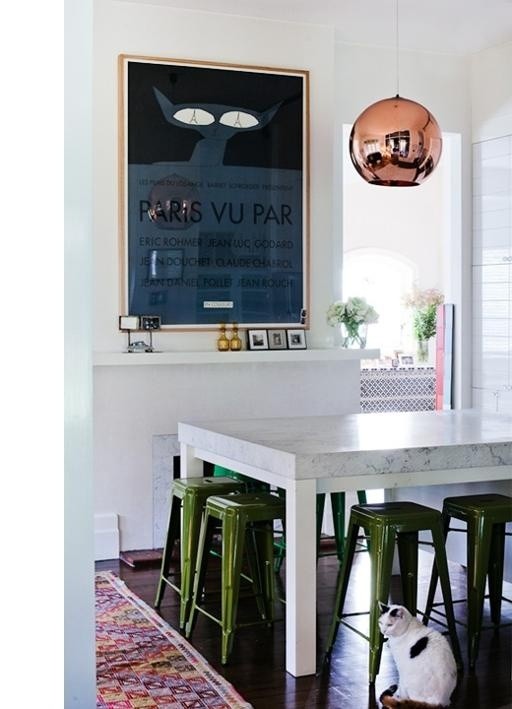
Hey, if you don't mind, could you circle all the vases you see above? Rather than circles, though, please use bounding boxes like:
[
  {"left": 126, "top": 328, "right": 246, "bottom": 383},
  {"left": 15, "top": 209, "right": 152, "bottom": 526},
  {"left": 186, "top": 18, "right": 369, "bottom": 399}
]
[
  {"left": 417, "top": 339, "right": 428, "bottom": 364},
  {"left": 338, "top": 323, "right": 368, "bottom": 349}
]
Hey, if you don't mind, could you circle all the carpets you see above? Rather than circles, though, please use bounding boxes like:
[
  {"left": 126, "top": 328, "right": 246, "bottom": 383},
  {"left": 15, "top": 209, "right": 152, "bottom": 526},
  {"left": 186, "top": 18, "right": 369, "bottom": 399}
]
[
  {"left": 94, "top": 570, "right": 254, "bottom": 709},
  {"left": 120, "top": 534, "right": 340, "bottom": 568}
]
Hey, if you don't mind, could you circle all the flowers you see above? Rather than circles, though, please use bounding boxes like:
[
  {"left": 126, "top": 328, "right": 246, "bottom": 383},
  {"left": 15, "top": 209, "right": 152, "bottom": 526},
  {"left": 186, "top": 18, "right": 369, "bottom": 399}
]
[
  {"left": 400, "top": 289, "right": 445, "bottom": 356},
  {"left": 326, "top": 298, "right": 378, "bottom": 345}
]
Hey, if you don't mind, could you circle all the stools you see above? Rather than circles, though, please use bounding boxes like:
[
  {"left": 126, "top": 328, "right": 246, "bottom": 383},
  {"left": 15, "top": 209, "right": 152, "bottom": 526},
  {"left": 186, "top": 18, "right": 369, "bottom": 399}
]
[
  {"left": 319, "top": 500, "right": 467, "bottom": 680},
  {"left": 421, "top": 488, "right": 512, "bottom": 675},
  {"left": 183, "top": 490, "right": 297, "bottom": 668},
  {"left": 313, "top": 488, "right": 381, "bottom": 577},
  {"left": 154, "top": 474, "right": 268, "bottom": 631}
]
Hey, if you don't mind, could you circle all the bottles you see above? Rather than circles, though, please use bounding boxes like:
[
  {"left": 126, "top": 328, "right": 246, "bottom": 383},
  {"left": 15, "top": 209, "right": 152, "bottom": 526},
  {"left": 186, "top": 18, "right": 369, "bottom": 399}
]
[{"left": 218, "top": 326, "right": 241, "bottom": 352}]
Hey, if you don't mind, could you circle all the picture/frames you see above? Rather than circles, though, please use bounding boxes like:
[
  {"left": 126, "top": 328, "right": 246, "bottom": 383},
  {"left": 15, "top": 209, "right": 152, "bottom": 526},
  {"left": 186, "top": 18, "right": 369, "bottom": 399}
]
[
  {"left": 267, "top": 328, "right": 288, "bottom": 350},
  {"left": 247, "top": 329, "right": 269, "bottom": 350},
  {"left": 287, "top": 328, "right": 307, "bottom": 350},
  {"left": 116, "top": 52, "right": 310, "bottom": 330},
  {"left": 401, "top": 356, "right": 413, "bottom": 368}
]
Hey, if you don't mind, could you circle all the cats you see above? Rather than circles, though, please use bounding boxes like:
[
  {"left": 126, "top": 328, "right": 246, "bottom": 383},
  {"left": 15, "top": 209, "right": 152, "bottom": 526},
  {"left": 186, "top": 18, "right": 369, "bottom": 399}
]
[{"left": 374, "top": 601, "right": 458, "bottom": 709}]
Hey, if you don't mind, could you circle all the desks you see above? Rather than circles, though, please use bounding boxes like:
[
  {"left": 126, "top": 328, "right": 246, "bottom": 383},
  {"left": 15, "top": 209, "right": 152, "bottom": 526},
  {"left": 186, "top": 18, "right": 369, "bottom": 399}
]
[{"left": 177, "top": 408, "right": 511, "bottom": 679}]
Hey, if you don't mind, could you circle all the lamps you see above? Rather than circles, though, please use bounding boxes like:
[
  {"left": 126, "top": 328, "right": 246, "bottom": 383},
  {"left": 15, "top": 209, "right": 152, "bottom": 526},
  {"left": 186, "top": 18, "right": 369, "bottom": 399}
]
[{"left": 347, "top": 0, "right": 441, "bottom": 186}]
